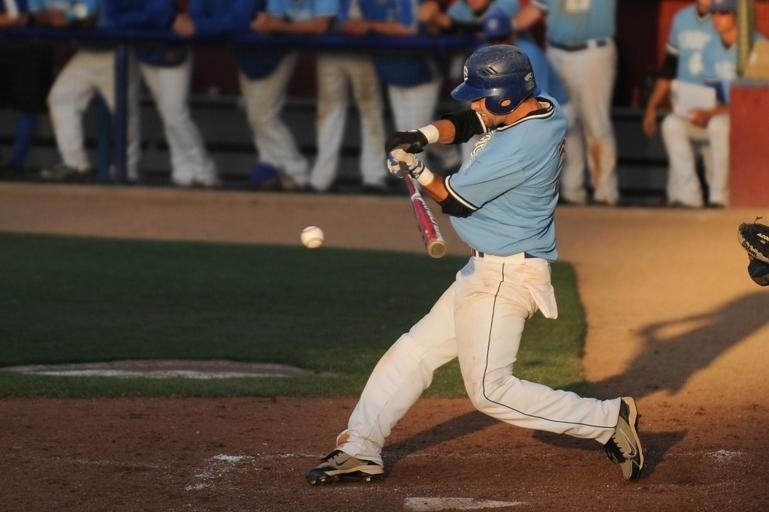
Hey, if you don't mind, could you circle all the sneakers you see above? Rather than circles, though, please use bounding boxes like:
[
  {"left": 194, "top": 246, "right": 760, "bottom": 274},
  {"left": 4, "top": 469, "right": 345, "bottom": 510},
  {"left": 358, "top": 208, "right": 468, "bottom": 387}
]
[
  {"left": 306, "top": 449, "right": 385, "bottom": 486},
  {"left": 603, "top": 396, "right": 645, "bottom": 482},
  {"left": 40, "top": 164, "right": 93, "bottom": 185}
]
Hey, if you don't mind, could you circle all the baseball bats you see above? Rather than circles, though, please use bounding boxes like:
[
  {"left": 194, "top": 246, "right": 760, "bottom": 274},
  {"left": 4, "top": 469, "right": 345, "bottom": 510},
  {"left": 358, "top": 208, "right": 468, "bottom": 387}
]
[{"left": 399, "top": 161, "right": 448, "bottom": 259}]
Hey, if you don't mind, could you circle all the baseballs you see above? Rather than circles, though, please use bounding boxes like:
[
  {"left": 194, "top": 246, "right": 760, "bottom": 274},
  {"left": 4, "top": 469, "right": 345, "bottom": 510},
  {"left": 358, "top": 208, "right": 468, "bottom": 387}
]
[{"left": 301, "top": 226, "right": 324, "bottom": 249}]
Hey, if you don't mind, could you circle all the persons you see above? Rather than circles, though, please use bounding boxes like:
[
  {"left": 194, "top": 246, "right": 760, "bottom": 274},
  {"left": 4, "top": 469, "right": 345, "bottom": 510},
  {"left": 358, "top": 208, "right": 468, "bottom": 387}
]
[{"left": 305, "top": 43, "right": 645, "bottom": 486}]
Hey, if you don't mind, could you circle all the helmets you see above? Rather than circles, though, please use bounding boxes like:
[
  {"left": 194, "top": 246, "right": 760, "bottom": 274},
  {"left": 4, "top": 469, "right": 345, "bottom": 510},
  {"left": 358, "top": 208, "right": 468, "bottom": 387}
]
[{"left": 450, "top": 44, "right": 537, "bottom": 116}]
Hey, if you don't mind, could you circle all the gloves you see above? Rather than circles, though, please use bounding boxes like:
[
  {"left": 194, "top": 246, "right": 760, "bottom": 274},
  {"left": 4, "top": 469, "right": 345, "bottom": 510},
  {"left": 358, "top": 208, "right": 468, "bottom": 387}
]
[
  {"left": 384, "top": 128, "right": 428, "bottom": 157},
  {"left": 386, "top": 148, "right": 425, "bottom": 181}
]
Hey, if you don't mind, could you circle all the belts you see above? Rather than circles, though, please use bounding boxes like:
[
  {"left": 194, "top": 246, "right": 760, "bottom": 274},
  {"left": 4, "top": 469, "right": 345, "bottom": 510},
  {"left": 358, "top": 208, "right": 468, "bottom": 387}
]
[
  {"left": 472, "top": 247, "right": 539, "bottom": 260},
  {"left": 550, "top": 37, "right": 610, "bottom": 53}
]
